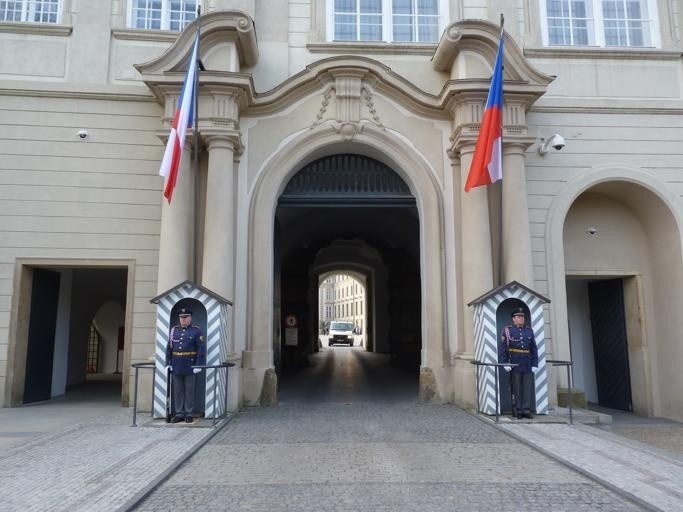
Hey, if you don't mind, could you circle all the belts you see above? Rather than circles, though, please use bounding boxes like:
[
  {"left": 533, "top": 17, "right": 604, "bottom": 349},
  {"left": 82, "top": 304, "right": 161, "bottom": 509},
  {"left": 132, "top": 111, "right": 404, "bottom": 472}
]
[
  {"left": 508, "top": 348, "right": 531, "bottom": 353},
  {"left": 171, "top": 351, "right": 197, "bottom": 355}
]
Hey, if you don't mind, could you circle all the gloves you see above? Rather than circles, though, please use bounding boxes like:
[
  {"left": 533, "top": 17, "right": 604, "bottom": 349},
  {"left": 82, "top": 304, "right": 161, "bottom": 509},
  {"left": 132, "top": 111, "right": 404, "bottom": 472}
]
[
  {"left": 532, "top": 367, "right": 538, "bottom": 372},
  {"left": 193, "top": 368, "right": 202, "bottom": 374},
  {"left": 165, "top": 365, "right": 173, "bottom": 374},
  {"left": 504, "top": 365, "right": 512, "bottom": 372}
]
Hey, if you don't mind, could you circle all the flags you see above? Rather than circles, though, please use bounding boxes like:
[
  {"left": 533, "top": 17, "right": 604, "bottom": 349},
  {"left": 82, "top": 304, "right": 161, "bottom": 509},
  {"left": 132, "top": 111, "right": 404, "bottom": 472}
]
[
  {"left": 455, "top": 22, "right": 508, "bottom": 195},
  {"left": 154, "top": 22, "right": 203, "bottom": 203}
]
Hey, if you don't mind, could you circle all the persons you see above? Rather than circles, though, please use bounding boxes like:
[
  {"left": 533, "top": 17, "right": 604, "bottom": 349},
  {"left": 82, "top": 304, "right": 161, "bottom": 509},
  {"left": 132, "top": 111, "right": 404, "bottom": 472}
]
[
  {"left": 497, "top": 307, "right": 538, "bottom": 420},
  {"left": 163, "top": 305, "right": 203, "bottom": 423}
]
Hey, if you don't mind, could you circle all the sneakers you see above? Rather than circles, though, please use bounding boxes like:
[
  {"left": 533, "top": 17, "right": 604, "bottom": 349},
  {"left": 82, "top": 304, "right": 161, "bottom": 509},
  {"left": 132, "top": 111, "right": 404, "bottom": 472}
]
[
  {"left": 516, "top": 409, "right": 533, "bottom": 419},
  {"left": 169, "top": 415, "right": 193, "bottom": 423}
]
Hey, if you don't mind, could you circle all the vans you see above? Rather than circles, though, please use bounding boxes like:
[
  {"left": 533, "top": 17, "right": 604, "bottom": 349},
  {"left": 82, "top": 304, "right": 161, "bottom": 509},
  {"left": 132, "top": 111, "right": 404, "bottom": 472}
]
[{"left": 327, "top": 319, "right": 355, "bottom": 348}]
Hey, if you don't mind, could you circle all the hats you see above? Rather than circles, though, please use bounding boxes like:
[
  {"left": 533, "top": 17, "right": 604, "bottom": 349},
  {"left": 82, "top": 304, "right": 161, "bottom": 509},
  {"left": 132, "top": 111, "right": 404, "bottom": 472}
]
[
  {"left": 512, "top": 307, "right": 526, "bottom": 316},
  {"left": 178, "top": 308, "right": 193, "bottom": 316}
]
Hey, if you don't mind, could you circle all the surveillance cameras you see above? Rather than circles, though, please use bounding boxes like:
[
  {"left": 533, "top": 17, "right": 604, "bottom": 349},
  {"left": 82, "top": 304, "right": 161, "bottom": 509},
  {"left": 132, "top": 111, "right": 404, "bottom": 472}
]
[
  {"left": 77, "top": 130, "right": 89, "bottom": 139},
  {"left": 589, "top": 227, "right": 597, "bottom": 234},
  {"left": 551, "top": 135, "right": 566, "bottom": 151}
]
